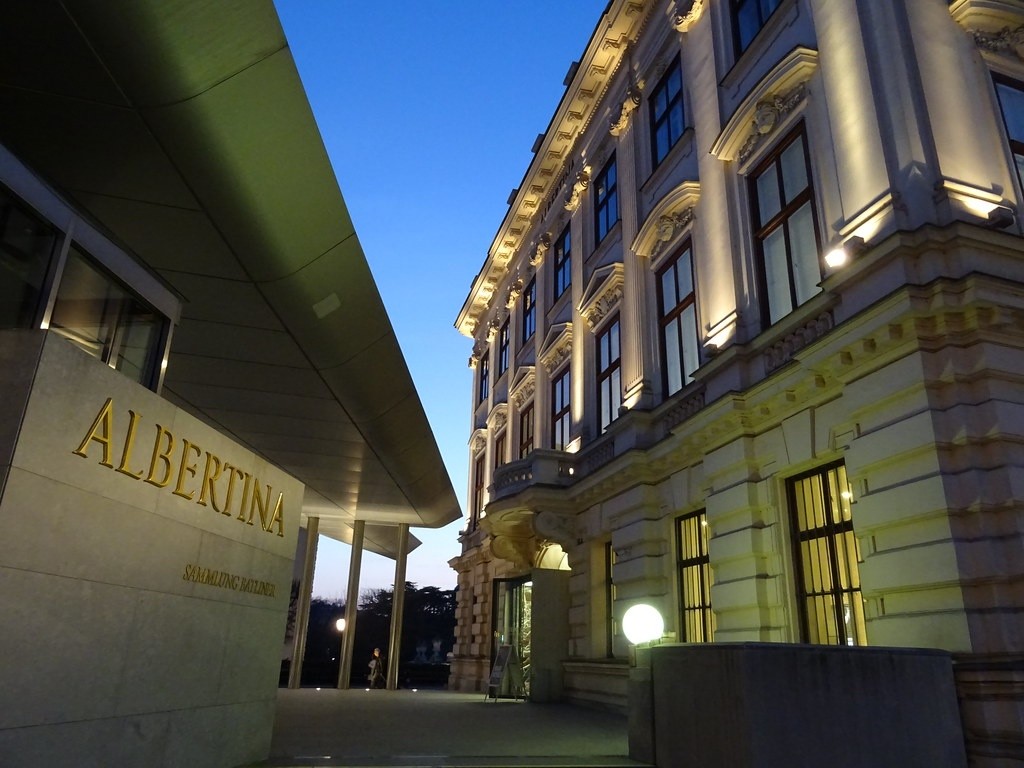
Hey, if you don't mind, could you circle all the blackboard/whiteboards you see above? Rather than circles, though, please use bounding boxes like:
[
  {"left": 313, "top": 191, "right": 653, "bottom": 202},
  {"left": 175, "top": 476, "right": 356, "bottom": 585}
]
[{"left": 489, "top": 646, "right": 513, "bottom": 686}]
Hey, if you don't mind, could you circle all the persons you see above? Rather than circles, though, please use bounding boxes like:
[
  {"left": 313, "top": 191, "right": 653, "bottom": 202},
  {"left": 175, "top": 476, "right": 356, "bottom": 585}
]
[{"left": 368, "top": 647, "right": 387, "bottom": 690}]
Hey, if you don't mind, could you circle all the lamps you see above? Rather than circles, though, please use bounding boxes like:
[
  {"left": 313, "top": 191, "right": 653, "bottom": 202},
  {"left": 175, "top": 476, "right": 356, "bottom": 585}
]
[
  {"left": 623, "top": 604, "right": 665, "bottom": 646},
  {"left": 988, "top": 207, "right": 1015, "bottom": 229},
  {"left": 825, "top": 236, "right": 864, "bottom": 268}
]
